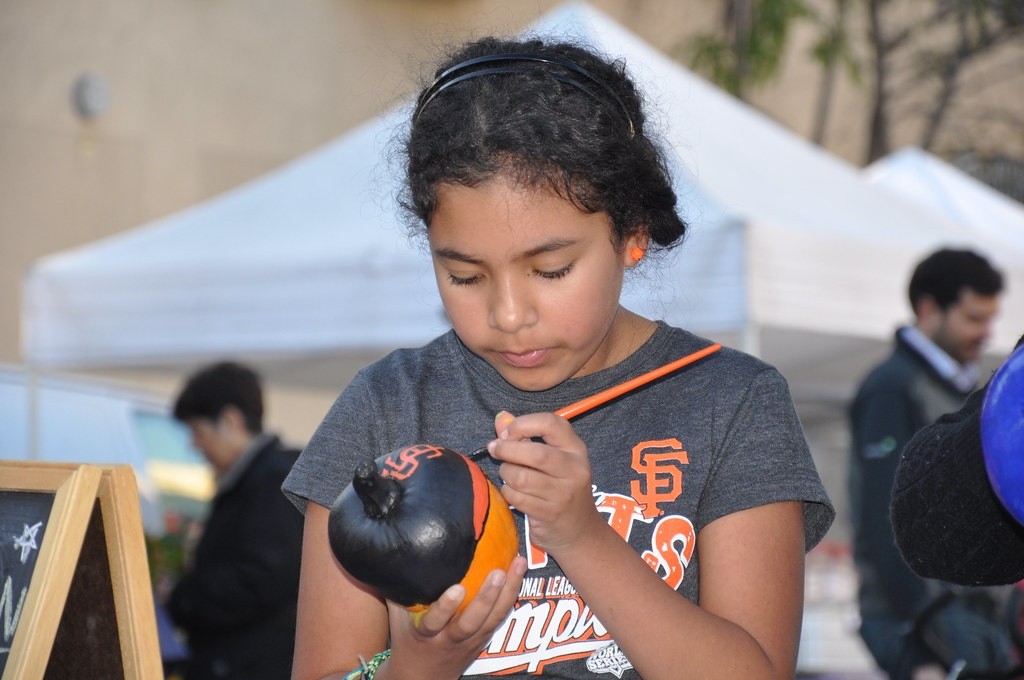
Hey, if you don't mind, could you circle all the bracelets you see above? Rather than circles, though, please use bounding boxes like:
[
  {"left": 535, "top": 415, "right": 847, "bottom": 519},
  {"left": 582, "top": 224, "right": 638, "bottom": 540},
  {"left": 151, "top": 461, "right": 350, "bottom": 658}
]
[{"left": 343, "top": 648, "right": 391, "bottom": 680}]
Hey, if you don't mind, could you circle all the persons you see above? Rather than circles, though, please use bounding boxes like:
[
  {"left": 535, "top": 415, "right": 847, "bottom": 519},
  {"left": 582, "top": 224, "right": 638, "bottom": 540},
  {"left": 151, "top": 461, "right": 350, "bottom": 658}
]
[
  {"left": 163, "top": 363, "right": 304, "bottom": 680},
  {"left": 845, "top": 250, "right": 1024, "bottom": 680},
  {"left": 280, "top": 38, "right": 837, "bottom": 680}
]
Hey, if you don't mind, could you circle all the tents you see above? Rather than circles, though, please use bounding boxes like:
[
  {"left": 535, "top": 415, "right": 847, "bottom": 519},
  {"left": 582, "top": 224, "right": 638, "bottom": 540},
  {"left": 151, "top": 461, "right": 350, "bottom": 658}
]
[{"left": 23, "top": 0, "right": 1024, "bottom": 464}]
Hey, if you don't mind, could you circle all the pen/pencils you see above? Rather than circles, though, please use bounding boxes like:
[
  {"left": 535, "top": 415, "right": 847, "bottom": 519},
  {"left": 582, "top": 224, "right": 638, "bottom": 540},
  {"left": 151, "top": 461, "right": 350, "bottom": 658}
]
[{"left": 945, "top": 658, "right": 969, "bottom": 680}]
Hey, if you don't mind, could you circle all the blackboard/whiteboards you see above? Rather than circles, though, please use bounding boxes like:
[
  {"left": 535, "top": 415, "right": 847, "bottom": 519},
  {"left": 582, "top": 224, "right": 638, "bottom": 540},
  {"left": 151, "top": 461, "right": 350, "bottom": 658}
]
[{"left": 0, "top": 461, "right": 173, "bottom": 680}]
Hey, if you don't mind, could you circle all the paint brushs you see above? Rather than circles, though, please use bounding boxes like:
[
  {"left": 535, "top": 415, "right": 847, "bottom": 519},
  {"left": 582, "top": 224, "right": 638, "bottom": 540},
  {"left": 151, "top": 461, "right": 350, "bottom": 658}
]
[{"left": 466, "top": 344, "right": 723, "bottom": 458}]
